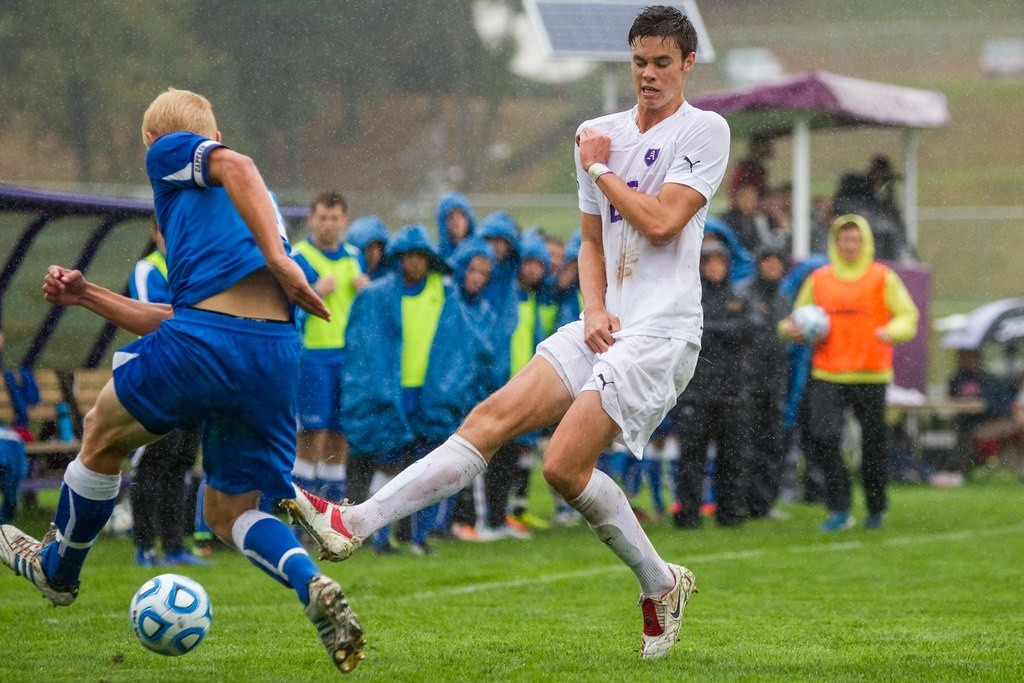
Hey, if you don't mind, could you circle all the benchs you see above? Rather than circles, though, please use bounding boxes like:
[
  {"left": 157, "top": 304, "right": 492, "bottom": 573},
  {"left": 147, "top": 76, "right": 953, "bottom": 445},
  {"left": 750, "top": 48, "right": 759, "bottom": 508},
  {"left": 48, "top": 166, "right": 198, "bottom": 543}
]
[{"left": 0, "top": 368, "right": 115, "bottom": 518}]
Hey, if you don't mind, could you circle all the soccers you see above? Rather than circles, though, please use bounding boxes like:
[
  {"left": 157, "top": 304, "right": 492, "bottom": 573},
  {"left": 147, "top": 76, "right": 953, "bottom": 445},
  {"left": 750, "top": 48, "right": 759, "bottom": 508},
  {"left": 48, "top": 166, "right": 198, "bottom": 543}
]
[{"left": 128, "top": 573, "right": 214, "bottom": 656}]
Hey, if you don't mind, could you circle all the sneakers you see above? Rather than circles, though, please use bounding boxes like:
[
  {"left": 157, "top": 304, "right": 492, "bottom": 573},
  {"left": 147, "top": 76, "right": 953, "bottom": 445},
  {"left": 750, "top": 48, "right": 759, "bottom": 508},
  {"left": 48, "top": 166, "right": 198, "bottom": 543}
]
[
  {"left": 0, "top": 524, "right": 81, "bottom": 606},
  {"left": 278, "top": 482, "right": 362, "bottom": 561},
  {"left": 304, "top": 576, "right": 366, "bottom": 674},
  {"left": 638, "top": 562, "right": 697, "bottom": 660}
]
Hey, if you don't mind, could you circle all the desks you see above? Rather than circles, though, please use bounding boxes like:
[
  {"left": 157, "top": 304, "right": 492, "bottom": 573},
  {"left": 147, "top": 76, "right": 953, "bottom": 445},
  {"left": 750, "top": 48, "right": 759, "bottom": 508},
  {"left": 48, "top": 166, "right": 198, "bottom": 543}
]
[{"left": 885, "top": 397, "right": 988, "bottom": 484}]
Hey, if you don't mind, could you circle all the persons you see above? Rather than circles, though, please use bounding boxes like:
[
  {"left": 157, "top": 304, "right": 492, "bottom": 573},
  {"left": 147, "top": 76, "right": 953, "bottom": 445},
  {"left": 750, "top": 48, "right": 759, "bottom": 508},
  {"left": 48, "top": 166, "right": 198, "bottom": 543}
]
[
  {"left": 944, "top": 342, "right": 1024, "bottom": 460},
  {"left": 776, "top": 214, "right": 919, "bottom": 532},
  {"left": 0, "top": 88, "right": 366, "bottom": 673},
  {"left": 591, "top": 137, "right": 904, "bottom": 528},
  {"left": 129, "top": 220, "right": 208, "bottom": 566},
  {"left": 278, "top": 5, "right": 731, "bottom": 660},
  {"left": 284, "top": 191, "right": 586, "bottom": 559}
]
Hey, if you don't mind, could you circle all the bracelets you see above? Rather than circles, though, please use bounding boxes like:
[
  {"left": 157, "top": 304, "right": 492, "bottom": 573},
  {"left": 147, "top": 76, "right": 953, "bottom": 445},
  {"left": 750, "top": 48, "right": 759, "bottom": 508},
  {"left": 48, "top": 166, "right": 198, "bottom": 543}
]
[{"left": 586, "top": 162, "right": 614, "bottom": 183}]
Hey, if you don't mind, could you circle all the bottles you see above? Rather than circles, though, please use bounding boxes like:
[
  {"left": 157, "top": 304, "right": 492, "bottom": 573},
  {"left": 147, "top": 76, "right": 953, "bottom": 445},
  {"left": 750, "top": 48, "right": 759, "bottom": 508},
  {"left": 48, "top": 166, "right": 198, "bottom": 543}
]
[{"left": 57, "top": 402, "right": 73, "bottom": 443}]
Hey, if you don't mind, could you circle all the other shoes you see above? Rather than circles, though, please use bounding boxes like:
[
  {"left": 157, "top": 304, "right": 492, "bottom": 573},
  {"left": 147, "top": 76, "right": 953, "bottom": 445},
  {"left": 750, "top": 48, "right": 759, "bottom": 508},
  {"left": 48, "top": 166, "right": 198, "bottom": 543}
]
[
  {"left": 376, "top": 502, "right": 889, "bottom": 557},
  {"left": 163, "top": 548, "right": 203, "bottom": 563},
  {"left": 137, "top": 549, "right": 164, "bottom": 566}
]
